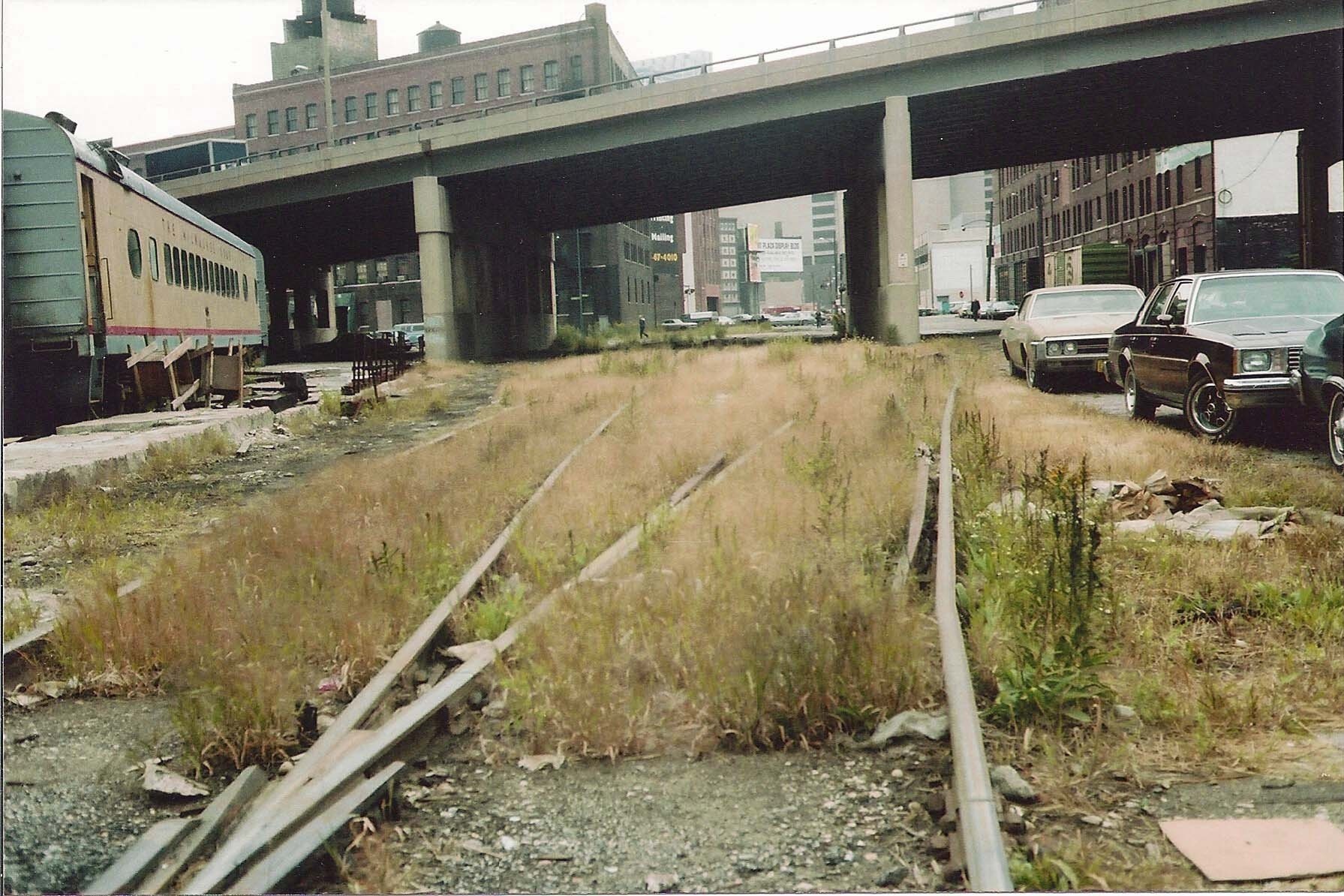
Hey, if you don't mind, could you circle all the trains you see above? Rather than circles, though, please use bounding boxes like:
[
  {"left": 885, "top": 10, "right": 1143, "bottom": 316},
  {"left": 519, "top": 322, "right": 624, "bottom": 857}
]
[{"left": 2, "top": 109, "right": 270, "bottom": 437}]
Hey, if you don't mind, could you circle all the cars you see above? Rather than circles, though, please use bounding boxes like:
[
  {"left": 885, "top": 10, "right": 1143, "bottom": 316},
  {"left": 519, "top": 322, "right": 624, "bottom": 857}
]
[
  {"left": 714, "top": 311, "right": 829, "bottom": 327},
  {"left": 368, "top": 330, "right": 411, "bottom": 351},
  {"left": 661, "top": 319, "right": 698, "bottom": 330},
  {"left": 919, "top": 307, "right": 938, "bottom": 316},
  {"left": 950, "top": 301, "right": 1019, "bottom": 320},
  {"left": 1109, "top": 266, "right": 1344, "bottom": 444},
  {"left": 1289, "top": 312, "right": 1344, "bottom": 479},
  {"left": 999, "top": 284, "right": 1146, "bottom": 394}
]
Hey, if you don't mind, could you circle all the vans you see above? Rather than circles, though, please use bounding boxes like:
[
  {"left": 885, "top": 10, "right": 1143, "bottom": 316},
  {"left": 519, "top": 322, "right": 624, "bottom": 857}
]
[{"left": 393, "top": 323, "right": 425, "bottom": 349}]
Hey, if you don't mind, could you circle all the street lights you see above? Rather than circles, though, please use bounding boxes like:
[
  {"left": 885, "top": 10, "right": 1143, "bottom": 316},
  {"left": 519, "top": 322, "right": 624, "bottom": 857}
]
[{"left": 296, "top": 0, "right": 337, "bottom": 147}]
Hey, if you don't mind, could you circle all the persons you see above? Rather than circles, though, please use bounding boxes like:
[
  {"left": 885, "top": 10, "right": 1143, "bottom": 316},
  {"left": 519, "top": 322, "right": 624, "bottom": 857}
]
[
  {"left": 815, "top": 310, "right": 822, "bottom": 329},
  {"left": 639, "top": 315, "right": 649, "bottom": 339},
  {"left": 971, "top": 299, "right": 980, "bottom": 322}
]
